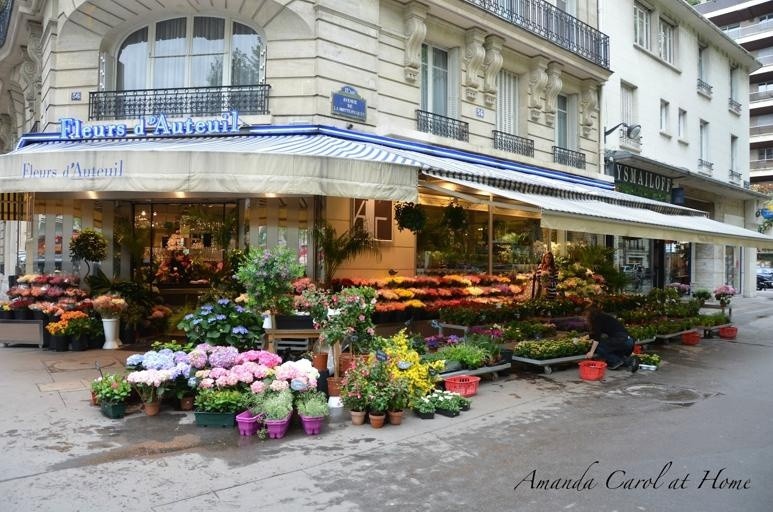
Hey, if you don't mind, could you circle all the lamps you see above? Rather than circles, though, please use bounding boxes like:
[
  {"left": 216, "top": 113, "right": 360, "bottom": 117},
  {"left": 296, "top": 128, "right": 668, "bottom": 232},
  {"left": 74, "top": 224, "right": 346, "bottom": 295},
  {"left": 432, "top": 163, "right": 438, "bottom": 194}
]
[{"left": 604, "top": 121, "right": 641, "bottom": 144}]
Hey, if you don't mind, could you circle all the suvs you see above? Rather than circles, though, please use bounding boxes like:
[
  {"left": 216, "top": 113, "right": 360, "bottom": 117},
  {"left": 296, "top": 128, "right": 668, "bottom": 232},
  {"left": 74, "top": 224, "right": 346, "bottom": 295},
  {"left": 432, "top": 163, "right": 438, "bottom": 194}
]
[{"left": 626, "top": 262, "right": 647, "bottom": 280}]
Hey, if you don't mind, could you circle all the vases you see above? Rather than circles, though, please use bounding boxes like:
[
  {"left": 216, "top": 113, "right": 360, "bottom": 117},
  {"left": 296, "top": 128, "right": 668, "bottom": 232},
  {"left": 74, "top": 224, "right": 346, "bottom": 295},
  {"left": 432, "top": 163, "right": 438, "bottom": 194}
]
[{"left": 194, "top": 410, "right": 404, "bottom": 439}]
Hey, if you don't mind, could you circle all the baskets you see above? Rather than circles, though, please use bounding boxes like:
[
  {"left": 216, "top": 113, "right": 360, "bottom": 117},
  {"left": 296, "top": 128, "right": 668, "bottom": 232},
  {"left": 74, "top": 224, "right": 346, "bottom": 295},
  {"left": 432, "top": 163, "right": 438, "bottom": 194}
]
[{"left": 444, "top": 375, "right": 481, "bottom": 398}]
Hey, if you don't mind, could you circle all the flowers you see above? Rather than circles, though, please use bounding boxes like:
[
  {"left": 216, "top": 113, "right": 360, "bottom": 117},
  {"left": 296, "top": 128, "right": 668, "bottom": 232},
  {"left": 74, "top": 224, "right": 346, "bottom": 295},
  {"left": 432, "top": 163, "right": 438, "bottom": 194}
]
[{"left": 0, "top": 234, "right": 737, "bottom": 419}]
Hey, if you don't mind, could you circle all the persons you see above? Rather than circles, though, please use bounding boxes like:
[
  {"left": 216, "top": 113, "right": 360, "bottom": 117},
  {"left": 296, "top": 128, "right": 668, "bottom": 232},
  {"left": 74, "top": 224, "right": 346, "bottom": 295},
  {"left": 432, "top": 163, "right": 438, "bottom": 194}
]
[
  {"left": 168, "top": 252, "right": 189, "bottom": 277},
  {"left": 580, "top": 302, "right": 640, "bottom": 374},
  {"left": 534, "top": 249, "right": 559, "bottom": 297}
]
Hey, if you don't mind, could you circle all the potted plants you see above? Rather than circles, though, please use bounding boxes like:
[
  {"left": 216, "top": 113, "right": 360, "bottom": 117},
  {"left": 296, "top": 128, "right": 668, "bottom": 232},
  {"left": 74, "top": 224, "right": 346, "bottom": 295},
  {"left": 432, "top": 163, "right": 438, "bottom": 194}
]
[
  {"left": 438, "top": 202, "right": 474, "bottom": 230},
  {"left": 393, "top": 202, "right": 428, "bottom": 231},
  {"left": 180, "top": 207, "right": 213, "bottom": 247}
]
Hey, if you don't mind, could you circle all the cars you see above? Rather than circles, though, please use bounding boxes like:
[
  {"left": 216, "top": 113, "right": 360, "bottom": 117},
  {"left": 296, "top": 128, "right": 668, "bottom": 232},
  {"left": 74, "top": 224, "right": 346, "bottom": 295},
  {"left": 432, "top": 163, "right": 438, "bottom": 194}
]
[
  {"left": 757, "top": 265, "right": 773, "bottom": 287},
  {"left": 619, "top": 266, "right": 636, "bottom": 279}
]
